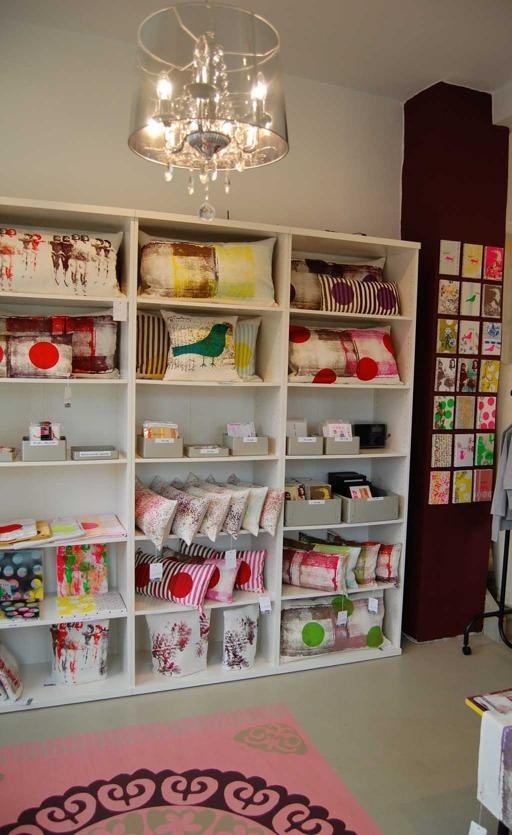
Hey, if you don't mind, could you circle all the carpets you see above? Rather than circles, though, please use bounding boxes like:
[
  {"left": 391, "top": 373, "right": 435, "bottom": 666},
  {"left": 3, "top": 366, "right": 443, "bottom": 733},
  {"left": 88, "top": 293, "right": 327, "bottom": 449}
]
[{"left": 0, "top": 704, "right": 385, "bottom": 834}]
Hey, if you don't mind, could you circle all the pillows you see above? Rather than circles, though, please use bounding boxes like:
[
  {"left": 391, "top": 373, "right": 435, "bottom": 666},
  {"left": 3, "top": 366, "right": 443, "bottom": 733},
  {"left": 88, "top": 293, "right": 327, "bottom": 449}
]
[
  {"left": 170, "top": 477, "right": 232, "bottom": 545},
  {"left": 137, "top": 308, "right": 263, "bottom": 382},
  {"left": 282, "top": 537, "right": 361, "bottom": 590},
  {"left": 147, "top": 474, "right": 210, "bottom": 547},
  {"left": 1, "top": 330, "right": 72, "bottom": 378},
  {"left": 207, "top": 475, "right": 270, "bottom": 539},
  {"left": 138, "top": 231, "right": 278, "bottom": 308},
  {"left": 156, "top": 310, "right": 242, "bottom": 381},
  {"left": 282, "top": 545, "right": 348, "bottom": 596},
  {"left": 228, "top": 473, "right": 285, "bottom": 539},
  {"left": 1, "top": 222, "right": 124, "bottom": 301},
  {"left": 148, "top": 612, "right": 211, "bottom": 680},
  {"left": 289, "top": 323, "right": 402, "bottom": 384},
  {"left": 161, "top": 544, "right": 243, "bottom": 604},
  {"left": 179, "top": 537, "right": 266, "bottom": 592},
  {"left": 185, "top": 471, "right": 250, "bottom": 541},
  {"left": 44, "top": 622, "right": 111, "bottom": 688},
  {"left": 327, "top": 528, "right": 402, "bottom": 589},
  {"left": 222, "top": 604, "right": 262, "bottom": 670},
  {"left": 281, "top": 591, "right": 386, "bottom": 655},
  {"left": 298, "top": 531, "right": 382, "bottom": 587},
  {"left": 134, "top": 474, "right": 177, "bottom": 555},
  {"left": 135, "top": 547, "right": 216, "bottom": 609},
  {"left": 320, "top": 273, "right": 402, "bottom": 314},
  {"left": 291, "top": 256, "right": 386, "bottom": 312},
  {"left": 0, "top": 311, "right": 122, "bottom": 378}
]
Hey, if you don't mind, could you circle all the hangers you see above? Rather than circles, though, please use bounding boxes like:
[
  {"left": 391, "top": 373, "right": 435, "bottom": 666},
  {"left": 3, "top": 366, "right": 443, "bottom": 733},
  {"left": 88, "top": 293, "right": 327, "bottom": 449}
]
[{"left": 460, "top": 385, "right": 512, "bottom": 657}]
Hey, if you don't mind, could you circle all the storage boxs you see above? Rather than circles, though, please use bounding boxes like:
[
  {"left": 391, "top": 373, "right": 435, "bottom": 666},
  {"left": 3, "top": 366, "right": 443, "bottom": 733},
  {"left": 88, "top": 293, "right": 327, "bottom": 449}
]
[
  {"left": 285, "top": 499, "right": 342, "bottom": 526},
  {"left": 333, "top": 490, "right": 400, "bottom": 523},
  {"left": 222, "top": 433, "right": 270, "bottom": 456},
  {"left": 322, "top": 431, "right": 361, "bottom": 455},
  {"left": 19, "top": 436, "right": 66, "bottom": 461},
  {"left": 289, "top": 435, "right": 324, "bottom": 458},
  {"left": 136, "top": 431, "right": 184, "bottom": 458}
]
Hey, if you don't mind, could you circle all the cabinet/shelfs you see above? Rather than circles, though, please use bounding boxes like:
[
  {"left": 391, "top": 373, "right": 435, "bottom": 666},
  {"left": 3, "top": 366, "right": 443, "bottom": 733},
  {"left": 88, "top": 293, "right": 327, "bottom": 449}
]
[
  {"left": 0, "top": 194, "right": 419, "bottom": 719},
  {"left": 401, "top": 82, "right": 512, "bottom": 644}
]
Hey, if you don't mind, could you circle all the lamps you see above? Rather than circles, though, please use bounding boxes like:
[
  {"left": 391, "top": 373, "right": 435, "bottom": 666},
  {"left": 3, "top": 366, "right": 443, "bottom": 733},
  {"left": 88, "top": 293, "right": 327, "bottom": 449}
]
[{"left": 129, "top": 0, "right": 289, "bottom": 221}]
